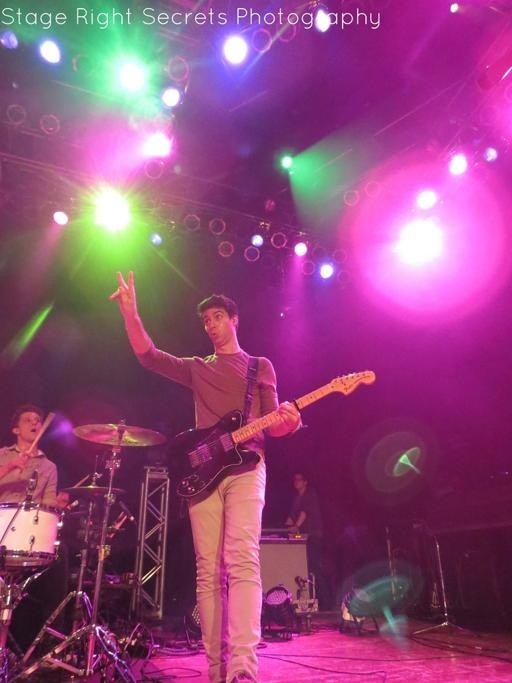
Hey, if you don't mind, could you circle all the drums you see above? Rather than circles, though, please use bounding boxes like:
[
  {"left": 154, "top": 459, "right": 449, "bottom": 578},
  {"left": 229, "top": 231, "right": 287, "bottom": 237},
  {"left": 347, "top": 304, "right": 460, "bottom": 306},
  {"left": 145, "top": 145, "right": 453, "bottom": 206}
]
[{"left": 0, "top": 502, "right": 65, "bottom": 572}]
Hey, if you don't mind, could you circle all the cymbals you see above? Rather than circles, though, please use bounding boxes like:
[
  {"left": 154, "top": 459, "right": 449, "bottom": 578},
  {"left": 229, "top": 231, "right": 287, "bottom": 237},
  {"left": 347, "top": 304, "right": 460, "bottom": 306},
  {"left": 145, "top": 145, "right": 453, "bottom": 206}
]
[
  {"left": 72, "top": 424, "right": 167, "bottom": 447},
  {"left": 63, "top": 486, "right": 123, "bottom": 494}
]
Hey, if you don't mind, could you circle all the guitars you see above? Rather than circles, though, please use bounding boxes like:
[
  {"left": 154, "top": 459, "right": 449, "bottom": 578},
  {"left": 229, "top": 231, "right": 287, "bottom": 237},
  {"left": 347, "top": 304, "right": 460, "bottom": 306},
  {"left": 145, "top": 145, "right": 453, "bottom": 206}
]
[{"left": 166, "top": 370, "right": 376, "bottom": 498}]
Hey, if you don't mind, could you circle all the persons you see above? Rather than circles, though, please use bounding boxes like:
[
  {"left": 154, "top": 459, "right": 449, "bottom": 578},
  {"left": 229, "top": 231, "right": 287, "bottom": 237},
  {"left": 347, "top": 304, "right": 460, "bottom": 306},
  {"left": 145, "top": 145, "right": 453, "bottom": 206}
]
[
  {"left": 284, "top": 470, "right": 321, "bottom": 600},
  {"left": 0, "top": 406, "right": 71, "bottom": 664},
  {"left": 109, "top": 271, "right": 302, "bottom": 683}
]
